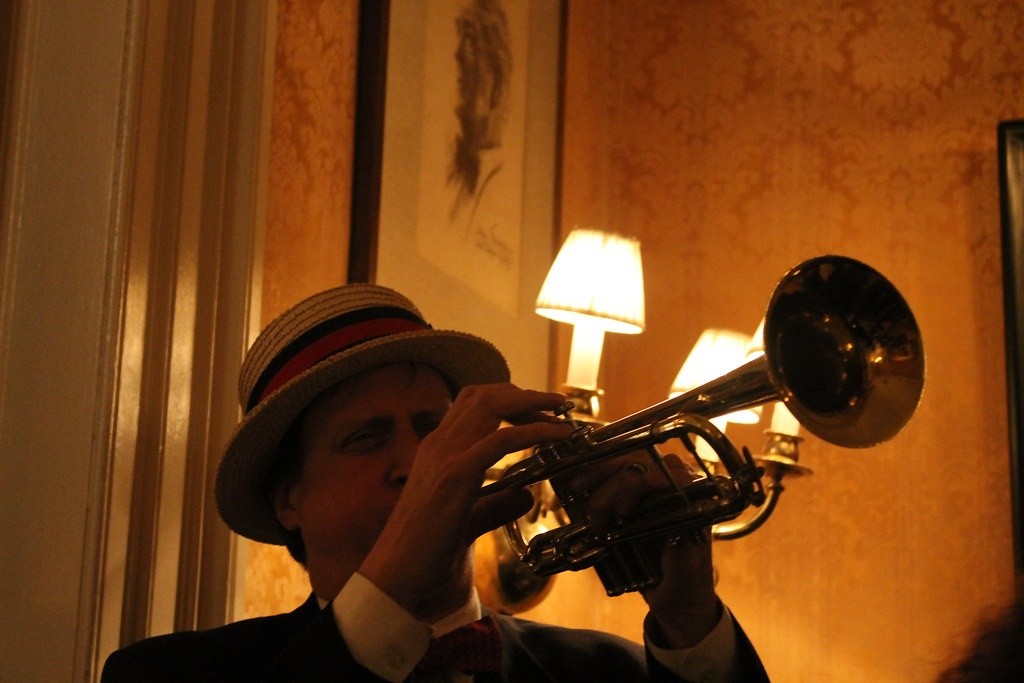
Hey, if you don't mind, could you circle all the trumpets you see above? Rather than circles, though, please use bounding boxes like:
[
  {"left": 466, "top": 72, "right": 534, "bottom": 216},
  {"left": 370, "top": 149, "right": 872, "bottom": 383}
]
[{"left": 469, "top": 255, "right": 925, "bottom": 578}]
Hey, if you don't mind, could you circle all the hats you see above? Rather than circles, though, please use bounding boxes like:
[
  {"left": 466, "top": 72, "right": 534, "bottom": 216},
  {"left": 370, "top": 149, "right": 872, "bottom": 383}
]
[{"left": 213, "top": 282, "right": 516, "bottom": 545}]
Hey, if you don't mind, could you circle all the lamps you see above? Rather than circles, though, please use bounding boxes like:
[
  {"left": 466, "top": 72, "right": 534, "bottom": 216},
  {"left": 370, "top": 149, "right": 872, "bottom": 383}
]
[{"left": 473, "top": 226, "right": 814, "bottom": 613}]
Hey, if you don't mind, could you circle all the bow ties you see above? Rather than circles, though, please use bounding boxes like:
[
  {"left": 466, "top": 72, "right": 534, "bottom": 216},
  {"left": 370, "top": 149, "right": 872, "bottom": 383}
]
[{"left": 405, "top": 613, "right": 505, "bottom": 683}]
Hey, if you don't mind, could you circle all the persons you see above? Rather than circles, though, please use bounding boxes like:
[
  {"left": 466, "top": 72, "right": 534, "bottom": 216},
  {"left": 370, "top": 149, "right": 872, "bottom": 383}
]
[{"left": 100, "top": 285, "right": 771, "bottom": 683}]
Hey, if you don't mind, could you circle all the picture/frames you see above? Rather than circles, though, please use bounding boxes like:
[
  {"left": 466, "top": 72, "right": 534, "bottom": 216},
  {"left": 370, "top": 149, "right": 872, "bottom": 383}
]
[{"left": 347, "top": 0, "right": 570, "bottom": 478}]
